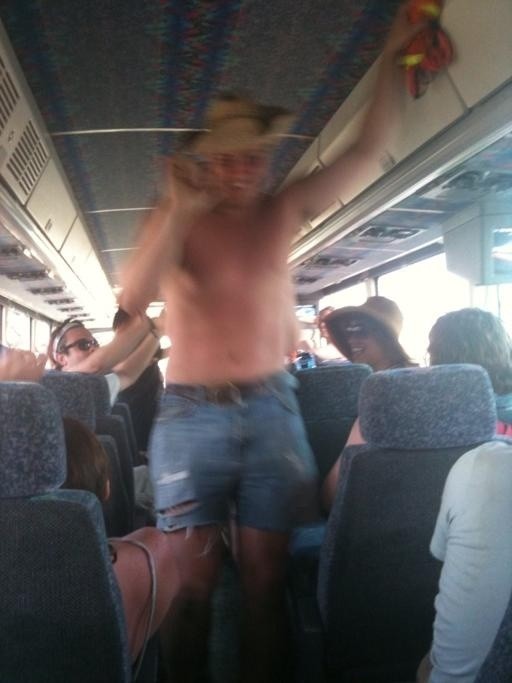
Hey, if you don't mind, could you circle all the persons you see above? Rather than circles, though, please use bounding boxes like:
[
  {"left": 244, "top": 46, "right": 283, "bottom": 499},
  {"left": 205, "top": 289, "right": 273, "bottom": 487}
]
[{"left": 120, "top": 0, "right": 426, "bottom": 681}]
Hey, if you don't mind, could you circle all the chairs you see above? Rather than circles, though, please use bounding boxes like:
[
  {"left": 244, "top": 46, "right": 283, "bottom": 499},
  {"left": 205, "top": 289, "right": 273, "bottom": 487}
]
[
  {"left": 291, "top": 365, "right": 512, "bottom": 683},
  {"left": 0, "top": 371, "right": 145, "bottom": 683}
]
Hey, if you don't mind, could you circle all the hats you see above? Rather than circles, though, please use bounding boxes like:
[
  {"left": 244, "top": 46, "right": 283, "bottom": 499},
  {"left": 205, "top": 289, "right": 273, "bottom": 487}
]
[
  {"left": 321, "top": 295, "right": 411, "bottom": 362},
  {"left": 175, "top": 92, "right": 298, "bottom": 162}
]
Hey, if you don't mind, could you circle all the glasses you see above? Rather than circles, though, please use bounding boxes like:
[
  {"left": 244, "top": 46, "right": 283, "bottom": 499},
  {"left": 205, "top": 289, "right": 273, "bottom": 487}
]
[
  {"left": 341, "top": 325, "right": 367, "bottom": 339},
  {"left": 61, "top": 337, "right": 99, "bottom": 352}
]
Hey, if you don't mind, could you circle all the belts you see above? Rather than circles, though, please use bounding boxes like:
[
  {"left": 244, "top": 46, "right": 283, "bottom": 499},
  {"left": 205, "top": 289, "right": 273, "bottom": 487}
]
[{"left": 181, "top": 384, "right": 264, "bottom": 402}]
